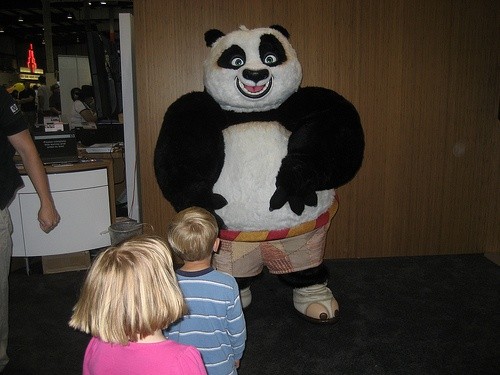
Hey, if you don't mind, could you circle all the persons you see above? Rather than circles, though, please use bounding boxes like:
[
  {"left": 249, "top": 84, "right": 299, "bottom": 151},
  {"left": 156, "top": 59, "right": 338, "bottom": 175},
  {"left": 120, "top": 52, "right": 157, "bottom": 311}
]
[
  {"left": 0, "top": 86, "right": 60, "bottom": 375},
  {"left": 49, "top": 83, "right": 62, "bottom": 116},
  {"left": 18, "top": 80, "right": 35, "bottom": 128},
  {"left": 32, "top": 85, "right": 38, "bottom": 104},
  {"left": 163, "top": 208, "right": 247, "bottom": 375},
  {"left": 3, "top": 83, "right": 18, "bottom": 102},
  {"left": 69, "top": 85, "right": 98, "bottom": 146},
  {"left": 37, "top": 75, "right": 50, "bottom": 124},
  {"left": 67, "top": 234, "right": 208, "bottom": 375}
]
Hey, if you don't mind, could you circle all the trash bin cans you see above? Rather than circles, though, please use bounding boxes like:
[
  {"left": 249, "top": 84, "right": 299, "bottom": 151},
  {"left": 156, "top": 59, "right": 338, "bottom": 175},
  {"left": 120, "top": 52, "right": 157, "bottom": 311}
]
[{"left": 109, "top": 223, "right": 145, "bottom": 246}]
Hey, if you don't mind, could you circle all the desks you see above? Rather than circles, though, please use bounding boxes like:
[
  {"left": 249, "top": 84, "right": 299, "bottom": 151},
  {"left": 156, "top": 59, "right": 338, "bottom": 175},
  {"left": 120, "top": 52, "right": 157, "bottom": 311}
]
[{"left": 7, "top": 149, "right": 126, "bottom": 275}]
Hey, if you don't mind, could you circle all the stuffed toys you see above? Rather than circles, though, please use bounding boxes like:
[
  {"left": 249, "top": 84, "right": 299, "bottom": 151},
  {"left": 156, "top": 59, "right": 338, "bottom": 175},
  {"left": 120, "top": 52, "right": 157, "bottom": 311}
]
[{"left": 154, "top": 24, "right": 365, "bottom": 324}]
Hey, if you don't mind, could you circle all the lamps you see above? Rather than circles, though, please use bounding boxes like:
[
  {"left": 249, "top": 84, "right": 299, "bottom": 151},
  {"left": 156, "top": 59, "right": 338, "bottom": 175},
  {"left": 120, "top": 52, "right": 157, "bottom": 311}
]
[
  {"left": 18, "top": 15, "right": 23, "bottom": 22},
  {"left": 0, "top": 28, "right": 4, "bottom": 33},
  {"left": 67, "top": 8, "right": 73, "bottom": 19}
]
